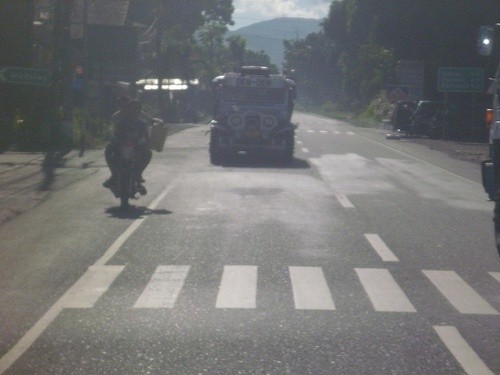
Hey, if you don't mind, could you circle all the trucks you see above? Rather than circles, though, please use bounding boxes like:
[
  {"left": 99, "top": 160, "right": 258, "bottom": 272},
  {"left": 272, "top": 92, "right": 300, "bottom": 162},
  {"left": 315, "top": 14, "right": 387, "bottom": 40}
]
[{"left": 208, "top": 66, "right": 298, "bottom": 167}]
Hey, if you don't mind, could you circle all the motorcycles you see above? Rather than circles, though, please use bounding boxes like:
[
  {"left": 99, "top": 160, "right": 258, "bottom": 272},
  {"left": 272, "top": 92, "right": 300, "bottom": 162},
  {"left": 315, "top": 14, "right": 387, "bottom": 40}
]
[{"left": 105, "top": 117, "right": 152, "bottom": 215}]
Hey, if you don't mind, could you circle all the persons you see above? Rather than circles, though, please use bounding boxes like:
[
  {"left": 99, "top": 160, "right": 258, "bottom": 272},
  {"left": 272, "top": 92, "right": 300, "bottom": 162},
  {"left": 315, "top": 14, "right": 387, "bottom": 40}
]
[
  {"left": 104, "top": 100, "right": 147, "bottom": 195},
  {"left": 110, "top": 96, "right": 157, "bottom": 184}
]
